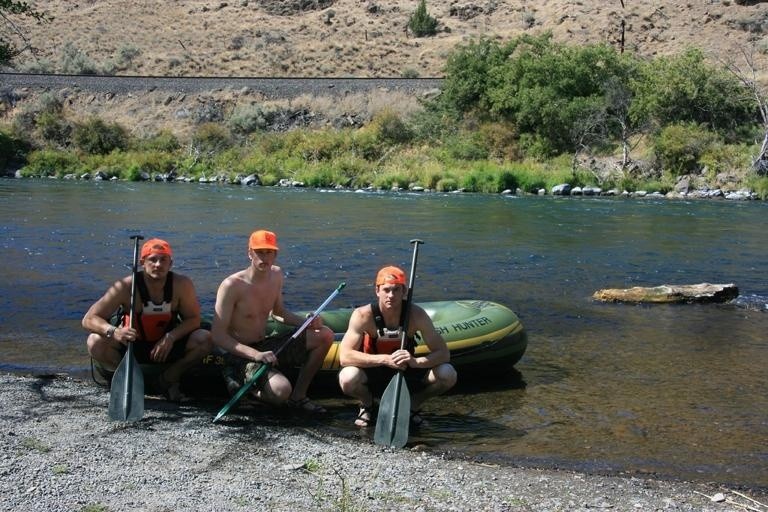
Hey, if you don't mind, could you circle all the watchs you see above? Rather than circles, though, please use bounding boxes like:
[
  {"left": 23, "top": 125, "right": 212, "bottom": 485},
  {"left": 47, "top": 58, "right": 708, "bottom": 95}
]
[{"left": 106, "top": 325, "right": 117, "bottom": 344}]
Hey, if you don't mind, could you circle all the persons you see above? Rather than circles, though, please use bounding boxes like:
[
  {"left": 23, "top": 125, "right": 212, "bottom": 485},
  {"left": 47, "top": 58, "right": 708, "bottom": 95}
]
[
  {"left": 211, "top": 227, "right": 334, "bottom": 418},
  {"left": 335, "top": 266, "right": 458, "bottom": 426},
  {"left": 81, "top": 238, "right": 212, "bottom": 402}
]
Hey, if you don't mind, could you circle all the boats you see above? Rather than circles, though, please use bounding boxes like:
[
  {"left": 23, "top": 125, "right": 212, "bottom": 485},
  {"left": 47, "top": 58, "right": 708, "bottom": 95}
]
[{"left": 91, "top": 299, "right": 529, "bottom": 389}]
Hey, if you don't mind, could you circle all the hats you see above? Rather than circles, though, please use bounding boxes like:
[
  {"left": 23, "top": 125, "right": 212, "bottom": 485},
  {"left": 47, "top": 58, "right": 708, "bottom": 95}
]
[
  {"left": 376, "top": 267, "right": 408, "bottom": 286},
  {"left": 141, "top": 239, "right": 173, "bottom": 259},
  {"left": 248, "top": 230, "right": 279, "bottom": 252}
]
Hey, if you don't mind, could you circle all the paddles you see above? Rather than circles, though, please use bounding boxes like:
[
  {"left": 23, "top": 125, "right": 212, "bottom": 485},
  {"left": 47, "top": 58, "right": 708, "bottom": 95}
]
[
  {"left": 108, "top": 234, "right": 144, "bottom": 423},
  {"left": 374, "top": 238, "right": 425, "bottom": 449},
  {"left": 212, "top": 282, "right": 347, "bottom": 424}
]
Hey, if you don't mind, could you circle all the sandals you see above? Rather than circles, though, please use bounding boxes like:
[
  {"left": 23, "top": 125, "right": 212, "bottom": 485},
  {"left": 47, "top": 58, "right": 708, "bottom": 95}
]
[
  {"left": 410, "top": 410, "right": 429, "bottom": 427},
  {"left": 164, "top": 389, "right": 196, "bottom": 405},
  {"left": 354, "top": 400, "right": 374, "bottom": 427},
  {"left": 287, "top": 396, "right": 327, "bottom": 413}
]
